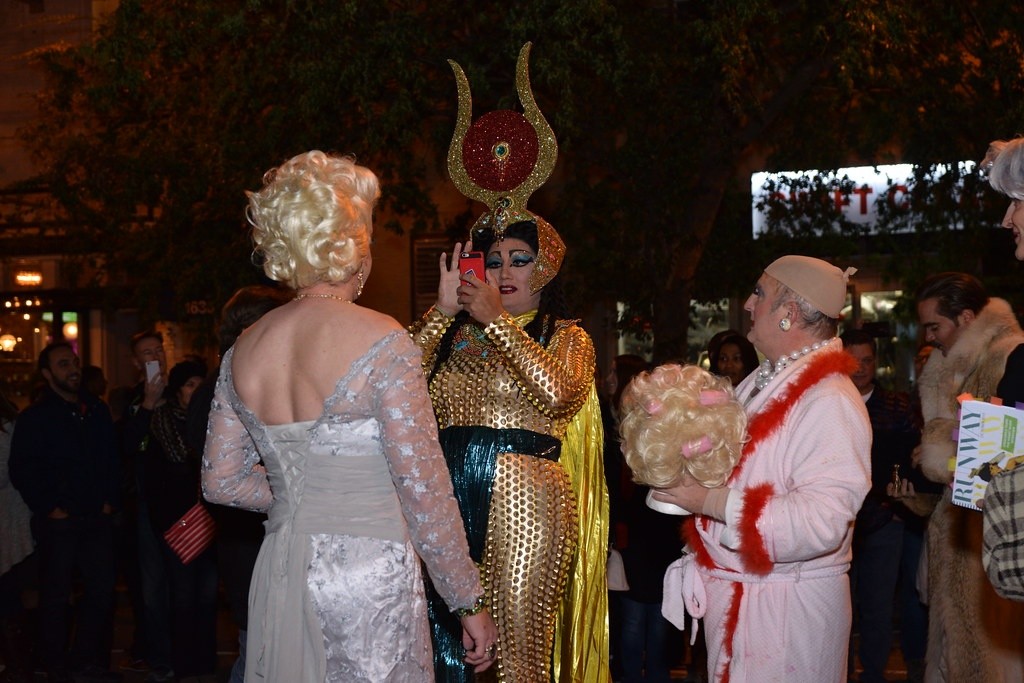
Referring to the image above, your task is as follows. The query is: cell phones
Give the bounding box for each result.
[144,360,160,385]
[457,250,485,297]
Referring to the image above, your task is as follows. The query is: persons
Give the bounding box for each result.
[201,150,500,683]
[841,330,930,683]
[596,330,760,683]
[408,209,610,683]
[886,137,1024,683]
[0,287,289,683]
[652,255,873,683]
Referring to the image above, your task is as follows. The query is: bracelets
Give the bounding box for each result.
[457,596,486,616]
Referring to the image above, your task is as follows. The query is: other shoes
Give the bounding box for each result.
[28,646,75,683]
[144,663,175,683]
[63,653,122,683]
[118,655,152,672]
[113,573,128,592]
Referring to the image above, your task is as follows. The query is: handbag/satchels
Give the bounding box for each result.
[162,502,220,567]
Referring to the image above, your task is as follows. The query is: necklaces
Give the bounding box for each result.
[753,337,836,389]
[295,294,352,303]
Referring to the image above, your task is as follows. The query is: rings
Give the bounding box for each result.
[486,641,497,659]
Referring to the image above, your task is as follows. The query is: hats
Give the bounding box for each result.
[184,354,209,378]
[169,362,202,390]
[764,255,859,319]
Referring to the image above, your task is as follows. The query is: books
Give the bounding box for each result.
[952,400,1024,512]
